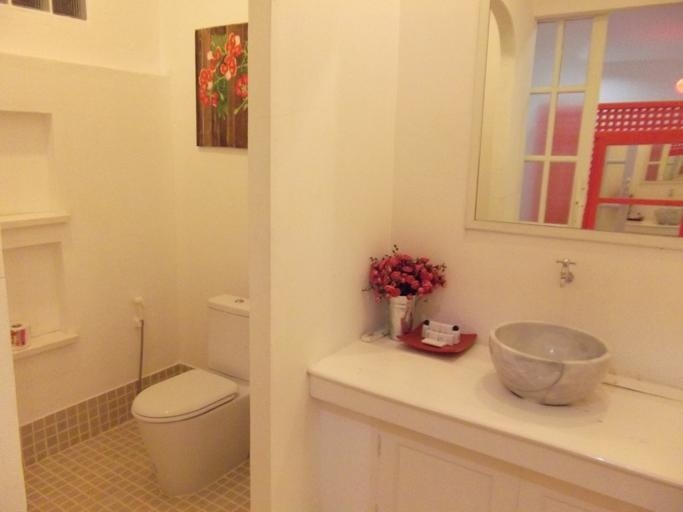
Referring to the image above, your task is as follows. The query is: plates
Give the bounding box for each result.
[397,321,478,356]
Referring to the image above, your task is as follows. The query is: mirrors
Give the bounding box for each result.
[462,0,682,257]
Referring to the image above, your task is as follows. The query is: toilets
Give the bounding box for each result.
[132,292,250,494]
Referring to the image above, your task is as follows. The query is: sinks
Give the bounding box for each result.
[489,319,613,405]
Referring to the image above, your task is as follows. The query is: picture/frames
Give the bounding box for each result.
[194,23,248,151]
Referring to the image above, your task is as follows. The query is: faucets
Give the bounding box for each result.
[557,258,578,288]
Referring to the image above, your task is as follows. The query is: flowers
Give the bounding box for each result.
[360,242,447,305]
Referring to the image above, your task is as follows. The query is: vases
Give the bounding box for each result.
[389,295,415,342]
